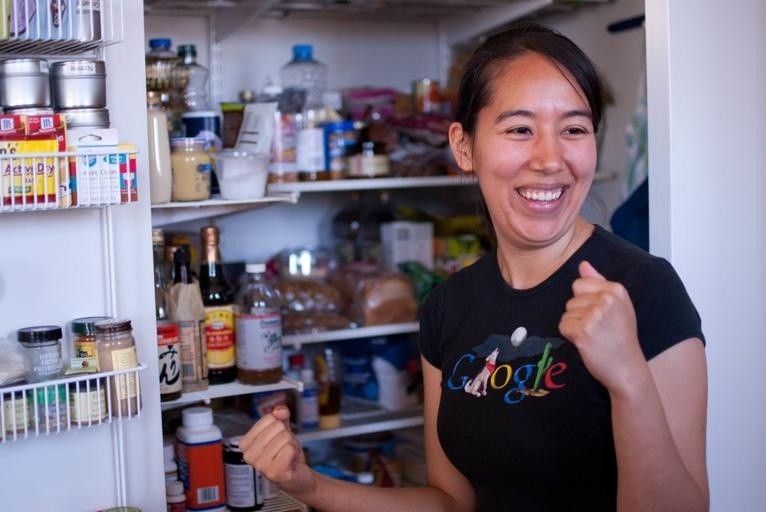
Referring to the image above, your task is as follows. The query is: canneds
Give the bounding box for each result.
[73,316,99,375]
[96,319,142,417]
[170,138,212,203]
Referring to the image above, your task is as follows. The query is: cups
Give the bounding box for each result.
[212,148,272,199]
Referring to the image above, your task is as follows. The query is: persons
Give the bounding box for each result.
[235,21,712,512]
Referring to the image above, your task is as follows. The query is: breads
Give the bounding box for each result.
[353,276,419,325]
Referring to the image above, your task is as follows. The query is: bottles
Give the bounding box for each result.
[237,261,284,386]
[152,222,184,404]
[218,43,391,179]
[284,343,345,431]
[144,36,220,202]
[197,227,238,384]
[168,237,210,393]
[162,407,226,510]
[0,313,141,431]
